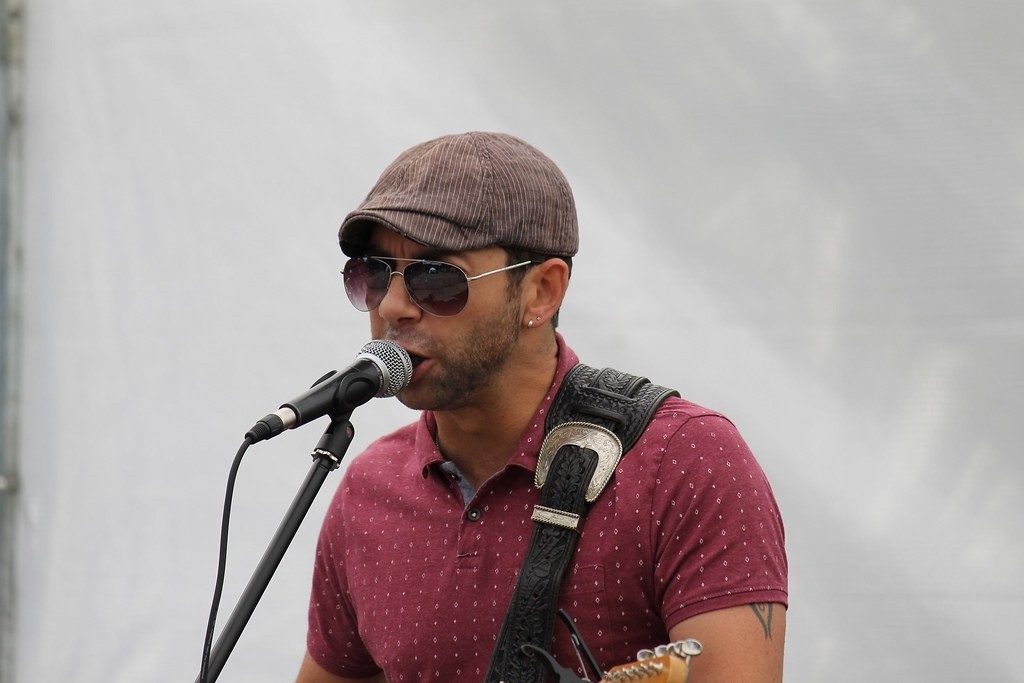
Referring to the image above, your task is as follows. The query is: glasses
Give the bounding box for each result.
[343,255,542,318]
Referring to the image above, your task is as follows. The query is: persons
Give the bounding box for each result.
[297,130,790,683]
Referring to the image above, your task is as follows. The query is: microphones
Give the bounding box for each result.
[245,340,413,444]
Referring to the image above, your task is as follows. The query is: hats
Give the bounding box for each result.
[337,130,579,257]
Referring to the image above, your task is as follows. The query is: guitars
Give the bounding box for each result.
[596,637,704,683]
